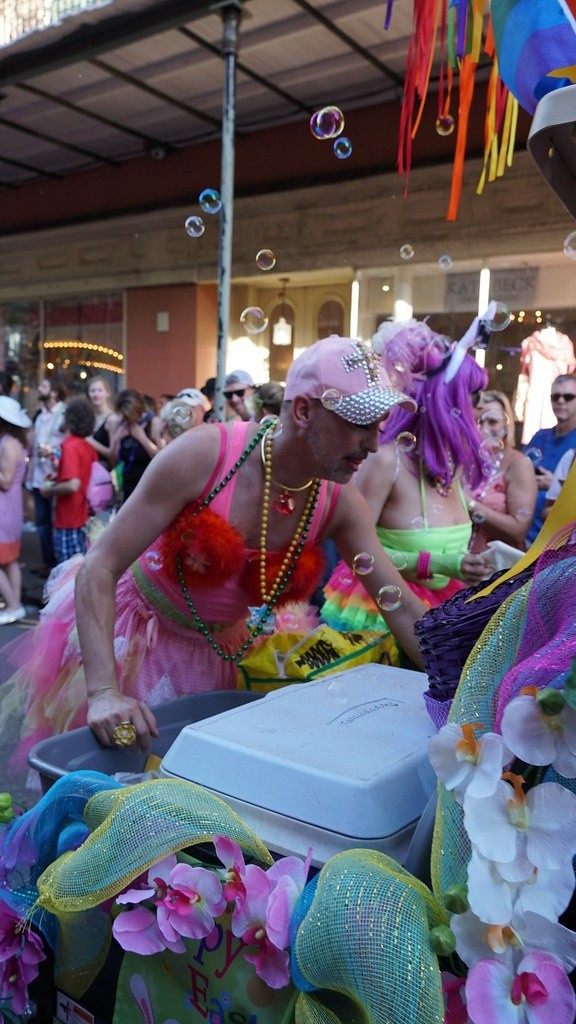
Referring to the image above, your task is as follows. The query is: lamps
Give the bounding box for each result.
[271,278,291,346]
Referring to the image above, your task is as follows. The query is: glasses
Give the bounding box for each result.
[550,393,576,402]
[224,386,252,400]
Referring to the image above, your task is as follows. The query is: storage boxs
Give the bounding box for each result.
[156,665,435,867]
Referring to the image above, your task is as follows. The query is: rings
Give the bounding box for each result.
[113,722,137,747]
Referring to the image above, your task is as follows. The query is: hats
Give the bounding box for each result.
[0,395,33,428]
[176,387,213,411]
[284,334,418,424]
[223,370,254,387]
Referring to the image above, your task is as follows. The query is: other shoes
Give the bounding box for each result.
[0,605,26,625]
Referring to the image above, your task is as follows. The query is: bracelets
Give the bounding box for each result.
[415,550,432,581]
[469,500,477,514]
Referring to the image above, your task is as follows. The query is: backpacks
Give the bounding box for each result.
[83,461,113,512]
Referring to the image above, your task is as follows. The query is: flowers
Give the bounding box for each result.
[0,682,576,1024]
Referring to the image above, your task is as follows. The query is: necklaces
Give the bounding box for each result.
[174,415,323,661]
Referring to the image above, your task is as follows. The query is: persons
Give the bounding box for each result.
[514,326,576,445]
[322,315,497,633]
[463,388,537,555]
[200,370,256,423]
[28,374,121,577]
[249,382,286,421]
[0,332,432,793]
[523,373,576,551]
[0,370,33,626]
[107,387,213,516]
[6,359,25,408]
